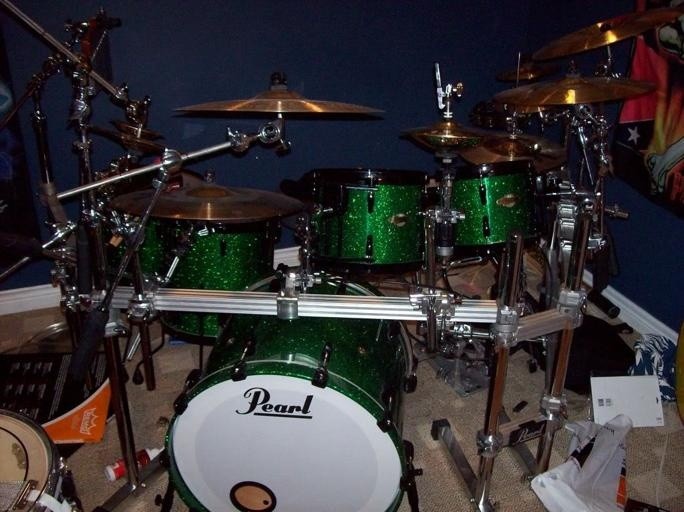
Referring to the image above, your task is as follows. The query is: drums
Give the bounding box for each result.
[431,159,540,260]
[139,218,274,337]
[166,271,409,512]
[306,169,426,270]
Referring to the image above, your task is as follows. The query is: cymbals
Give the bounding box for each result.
[530,8,681,61]
[398,122,487,145]
[108,182,304,220]
[505,64,568,84]
[460,131,566,169]
[496,80,654,105]
[175,91,386,115]
[89,119,169,154]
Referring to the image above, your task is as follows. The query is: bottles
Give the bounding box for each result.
[104,447,162,483]
